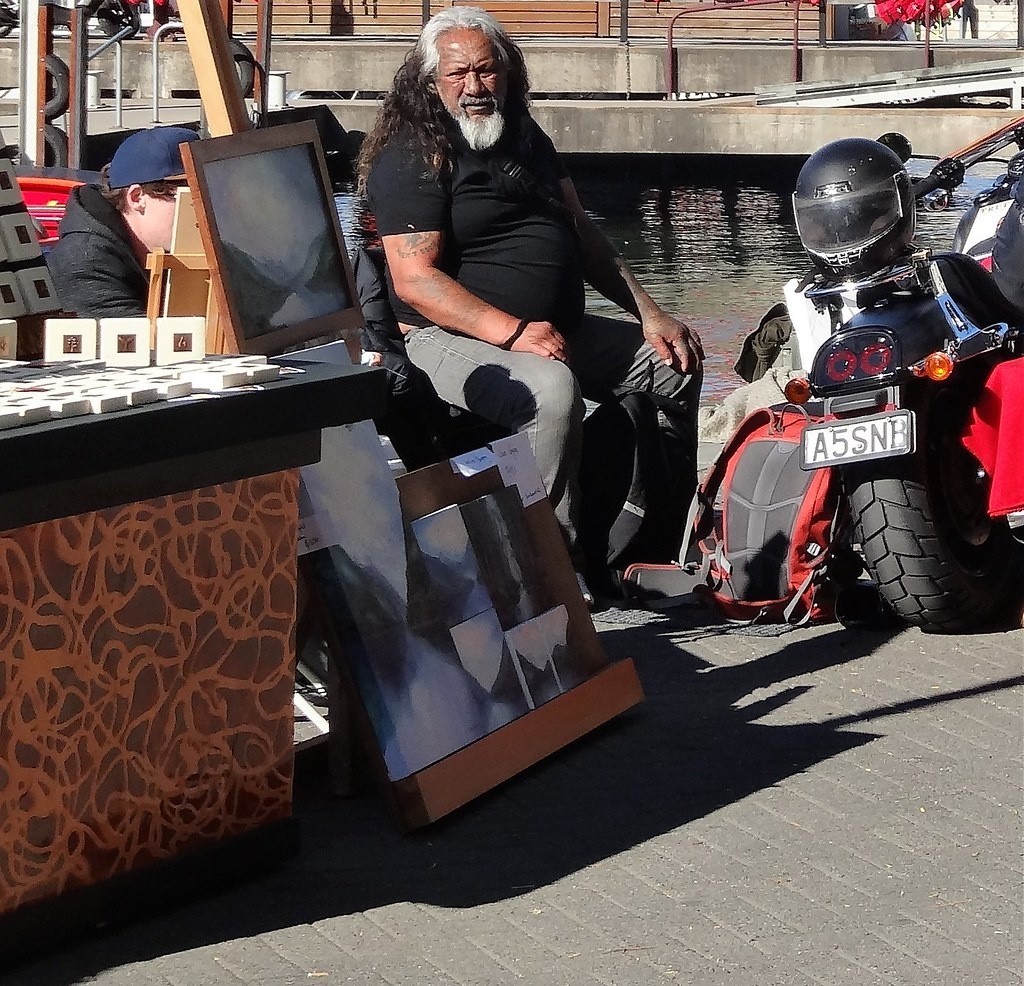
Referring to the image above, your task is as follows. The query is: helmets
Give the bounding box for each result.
[791,137,917,282]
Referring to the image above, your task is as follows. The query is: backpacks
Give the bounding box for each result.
[566,393,698,598]
[623,403,843,628]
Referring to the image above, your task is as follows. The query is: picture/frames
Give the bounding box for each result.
[178,119,367,358]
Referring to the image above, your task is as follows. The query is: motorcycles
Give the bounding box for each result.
[784,118,1023,634]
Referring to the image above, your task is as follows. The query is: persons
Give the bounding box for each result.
[46,128,200,318]
[358,6,706,605]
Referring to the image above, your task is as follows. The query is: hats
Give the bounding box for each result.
[108,126,201,189]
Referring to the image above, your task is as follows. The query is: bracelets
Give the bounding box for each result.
[501,317,530,351]
